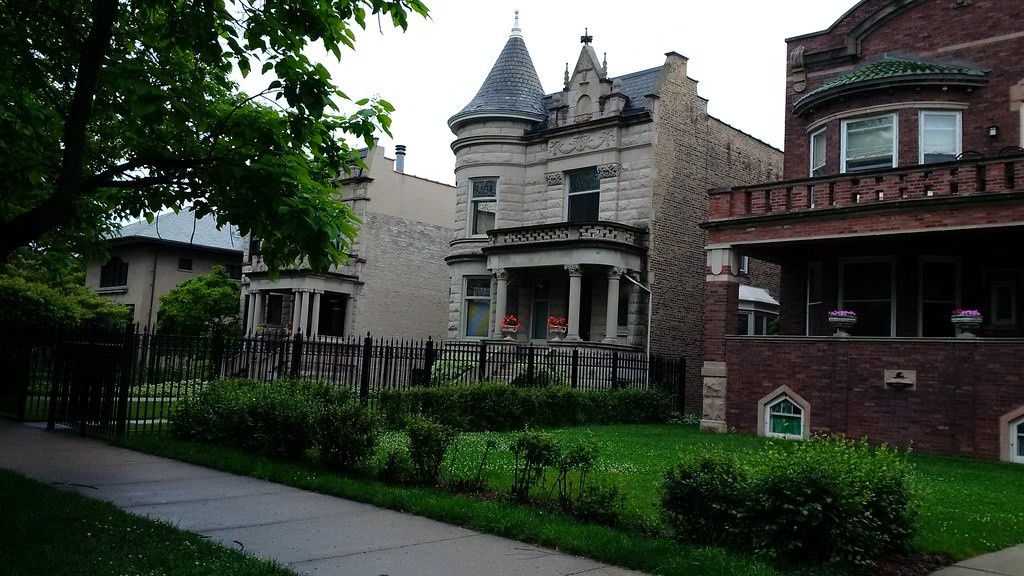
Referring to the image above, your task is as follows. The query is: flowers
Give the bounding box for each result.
[953,309,981,317]
[500,315,520,331]
[547,316,567,331]
[828,309,857,319]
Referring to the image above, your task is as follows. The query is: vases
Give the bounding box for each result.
[550,326,565,340]
[829,317,856,336]
[502,325,517,340]
[950,315,982,338]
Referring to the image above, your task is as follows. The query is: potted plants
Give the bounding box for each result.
[283,322,293,337]
[256,323,269,339]
